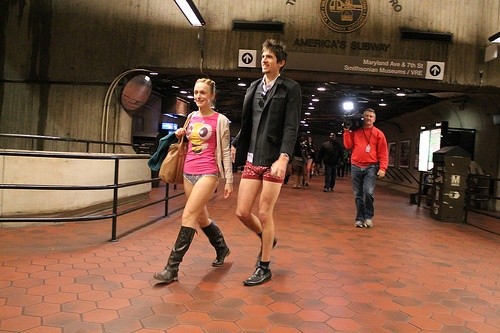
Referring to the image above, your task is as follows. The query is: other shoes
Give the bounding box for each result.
[306,182,308,187]
[331,188,334,191]
[292,184,300,188]
[302,181,304,185]
[324,188,328,191]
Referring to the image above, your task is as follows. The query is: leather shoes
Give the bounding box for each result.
[243,268,271,285]
[257,238,277,267]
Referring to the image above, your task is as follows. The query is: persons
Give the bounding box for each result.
[227,39,302,287]
[285,133,353,193]
[152,77,234,281]
[342,108,389,228]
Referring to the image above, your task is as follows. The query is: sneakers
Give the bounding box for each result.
[364,219,373,228]
[355,221,363,227]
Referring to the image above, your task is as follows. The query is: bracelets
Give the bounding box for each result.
[281,153,289,161]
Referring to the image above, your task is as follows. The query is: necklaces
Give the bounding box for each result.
[362,126,373,153]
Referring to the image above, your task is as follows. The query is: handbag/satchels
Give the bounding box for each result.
[158,116,193,184]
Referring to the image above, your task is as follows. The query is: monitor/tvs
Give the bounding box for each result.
[417,120,448,172]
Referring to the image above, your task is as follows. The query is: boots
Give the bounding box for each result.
[153,226,197,283]
[200,220,230,267]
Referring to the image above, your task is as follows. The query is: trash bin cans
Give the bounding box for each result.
[430,145,472,224]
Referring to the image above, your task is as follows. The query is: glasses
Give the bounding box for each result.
[259,91,268,109]
